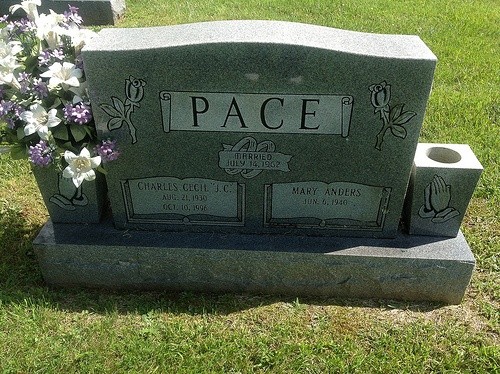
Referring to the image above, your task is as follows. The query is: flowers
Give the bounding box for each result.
[0,0,121,189]
